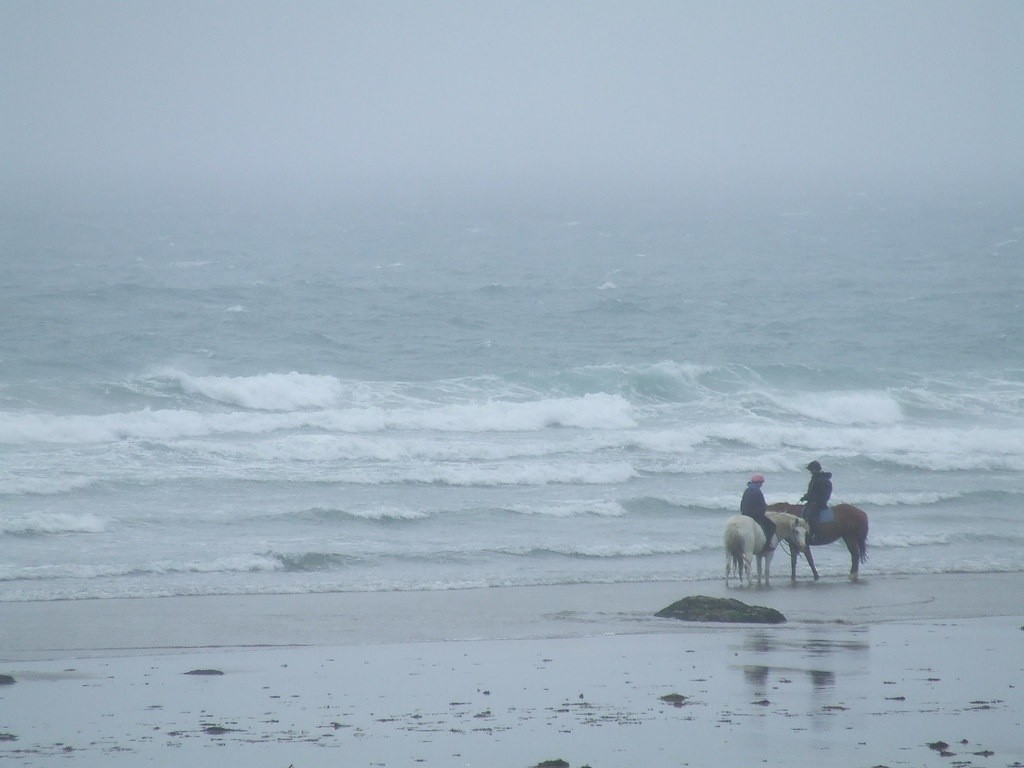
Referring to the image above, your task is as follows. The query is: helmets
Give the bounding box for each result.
[751,474,765,481]
[807,461,821,470]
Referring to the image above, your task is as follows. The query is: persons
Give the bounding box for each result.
[740,474,776,552]
[800,460,833,541]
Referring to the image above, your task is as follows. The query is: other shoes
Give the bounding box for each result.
[762,544,773,552]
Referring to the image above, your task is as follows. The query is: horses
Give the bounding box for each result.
[764,502,870,585]
[723,512,811,590]
